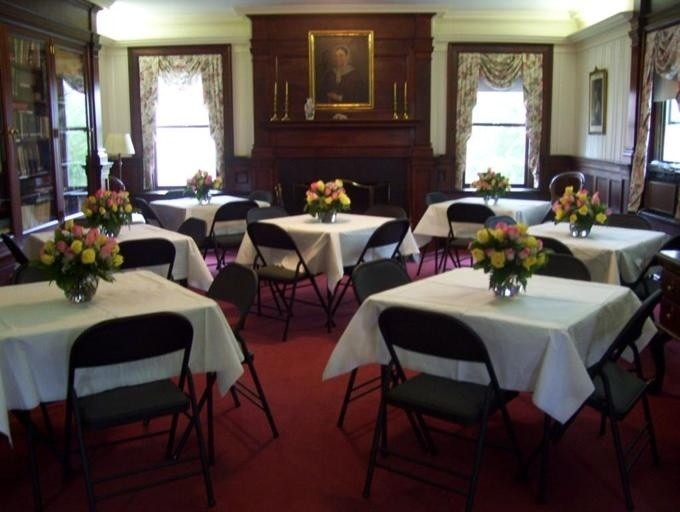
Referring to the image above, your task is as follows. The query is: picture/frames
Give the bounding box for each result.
[307,29,375,114]
[587,70,607,134]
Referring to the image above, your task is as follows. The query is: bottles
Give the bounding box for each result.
[305,98,315,120]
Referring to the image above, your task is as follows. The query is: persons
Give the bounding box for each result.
[323,47,362,103]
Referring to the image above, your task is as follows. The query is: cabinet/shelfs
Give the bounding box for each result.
[260,119,427,213]
[1,0,104,241]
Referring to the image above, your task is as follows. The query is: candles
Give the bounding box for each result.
[270,83,279,120]
[392,85,399,119]
[282,81,293,122]
[401,79,410,119]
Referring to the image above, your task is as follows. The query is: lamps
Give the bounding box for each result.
[104,132,135,180]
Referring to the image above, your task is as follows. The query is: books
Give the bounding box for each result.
[8,32,57,237]
